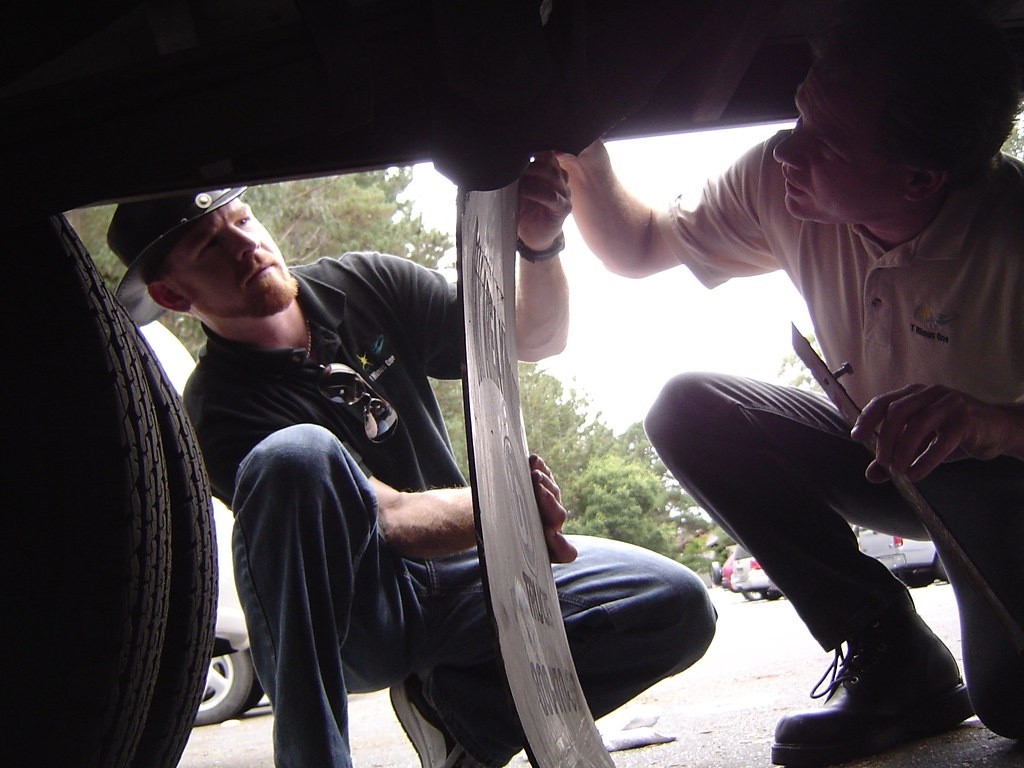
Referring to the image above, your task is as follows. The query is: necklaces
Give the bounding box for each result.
[302,330,312,358]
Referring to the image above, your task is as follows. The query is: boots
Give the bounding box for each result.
[772,585,975,765]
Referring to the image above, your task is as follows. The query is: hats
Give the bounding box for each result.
[106,186,249,326]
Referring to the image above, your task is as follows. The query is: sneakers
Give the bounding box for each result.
[389,674,481,768]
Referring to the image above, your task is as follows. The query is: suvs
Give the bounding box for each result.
[710,545,761,601]
[730,542,782,600]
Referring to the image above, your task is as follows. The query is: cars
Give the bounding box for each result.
[852,522,951,587]
[137,321,261,730]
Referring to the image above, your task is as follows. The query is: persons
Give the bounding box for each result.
[107,153,719,768]
[558,1,1024,768]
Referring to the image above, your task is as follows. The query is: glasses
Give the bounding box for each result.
[316,361,398,443]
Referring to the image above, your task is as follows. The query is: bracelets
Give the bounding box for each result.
[516,230,566,264]
